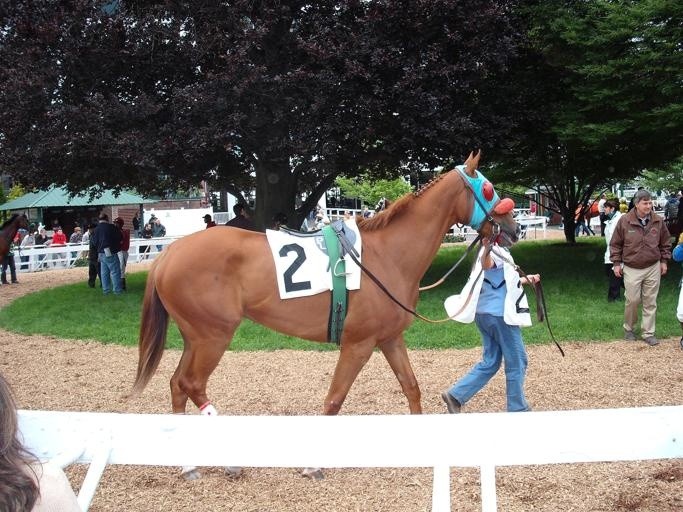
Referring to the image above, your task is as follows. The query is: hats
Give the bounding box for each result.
[113,218,124,224]
[202,214,210,218]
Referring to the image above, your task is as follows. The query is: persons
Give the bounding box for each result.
[0,373,44,511]
[561,188,683,348]
[441,236,541,412]
[269,212,298,233]
[0,204,254,296]
[530,200,537,226]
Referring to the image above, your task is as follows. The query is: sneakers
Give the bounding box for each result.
[442,393,460,414]
[625,331,636,341]
[645,336,659,345]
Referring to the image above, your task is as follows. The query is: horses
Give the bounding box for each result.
[375,197,392,212]
[562,199,599,236]
[0,212,35,289]
[120,149,521,481]
